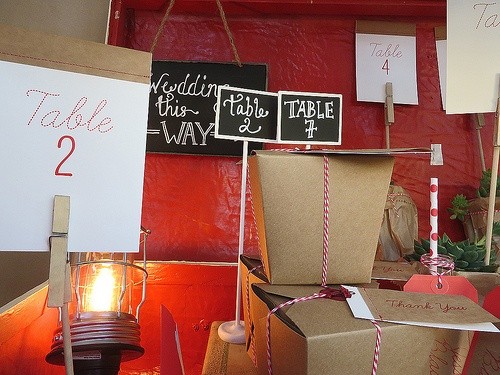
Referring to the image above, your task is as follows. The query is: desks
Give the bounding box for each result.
[200,319,251,374]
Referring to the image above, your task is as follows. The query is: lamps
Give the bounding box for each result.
[45,226,151,365]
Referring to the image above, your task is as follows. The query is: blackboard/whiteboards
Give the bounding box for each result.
[216,85,279,143]
[277,92,343,145]
[145,59,269,157]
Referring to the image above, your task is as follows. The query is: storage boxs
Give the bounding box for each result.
[247,149,395,285]
[249,283,475,375]
[240,254,380,368]
